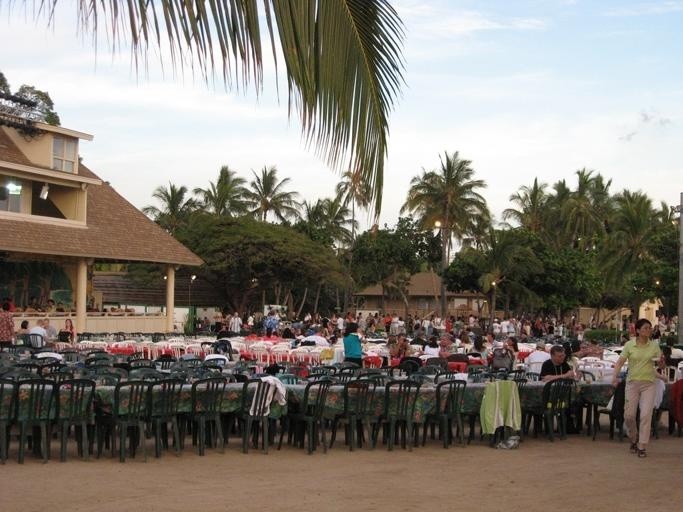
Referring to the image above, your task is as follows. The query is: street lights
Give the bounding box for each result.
[188,275,198,313]
[489,280,497,316]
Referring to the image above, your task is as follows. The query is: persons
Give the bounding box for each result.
[193,295,683,422]
[0,296,132,358]
[610,318,667,458]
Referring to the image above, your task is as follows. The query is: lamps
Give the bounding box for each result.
[39,182,49,200]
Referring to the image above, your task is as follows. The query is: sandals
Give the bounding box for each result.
[638,448,649,457]
[630,439,639,453]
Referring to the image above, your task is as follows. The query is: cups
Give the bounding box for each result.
[652,357,659,369]
[585,377,591,384]
